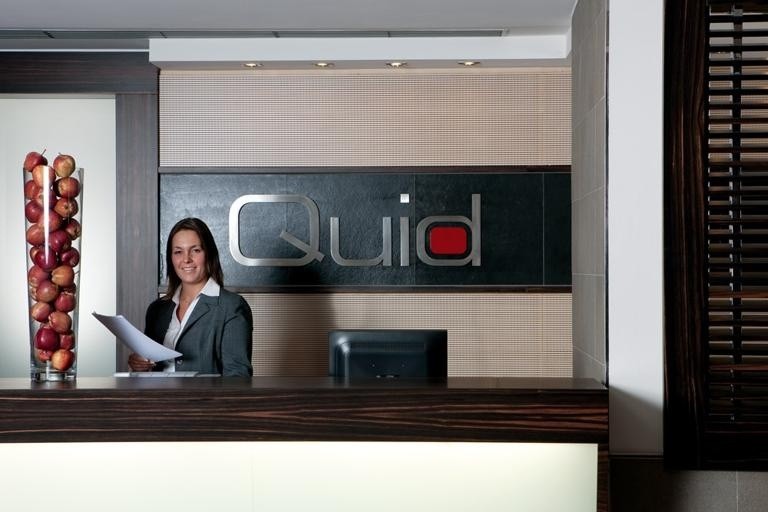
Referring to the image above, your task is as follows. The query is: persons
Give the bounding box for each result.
[126,216,255,376]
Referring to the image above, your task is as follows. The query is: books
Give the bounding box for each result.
[91,311,183,364]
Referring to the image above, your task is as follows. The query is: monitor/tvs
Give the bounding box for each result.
[326,329,448,379]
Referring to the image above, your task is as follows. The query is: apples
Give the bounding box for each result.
[23,149,82,372]
